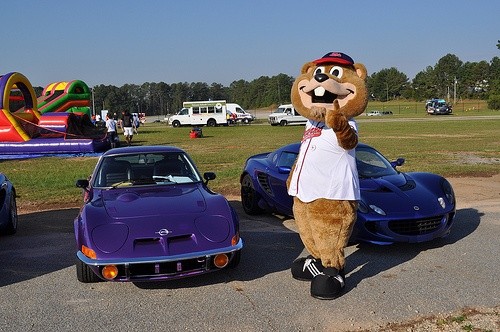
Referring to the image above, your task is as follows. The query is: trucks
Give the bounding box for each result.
[168,99,228,127]
[227,103,254,124]
[268,105,309,126]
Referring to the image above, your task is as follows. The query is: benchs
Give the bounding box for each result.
[129,162,155,180]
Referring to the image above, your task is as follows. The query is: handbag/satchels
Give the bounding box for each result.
[114,137,119,142]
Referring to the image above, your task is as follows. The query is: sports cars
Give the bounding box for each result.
[73,146,243,283]
[240,142,456,251]
[0,174,19,245]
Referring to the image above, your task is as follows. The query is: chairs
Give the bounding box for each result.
[98,160,130,187]
[153,157,188,182]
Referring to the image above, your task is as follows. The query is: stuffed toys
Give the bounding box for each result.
[286,52,369,300]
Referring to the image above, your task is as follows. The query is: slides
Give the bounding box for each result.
[14,80,91,113]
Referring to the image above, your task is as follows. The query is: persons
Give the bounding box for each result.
[96,112,100,121]
[106,114,118,143]
[120,109,137,146]
[227,113,237,123]
[189,126,202,138]
[91,114,95,120]
[134,115,138,129]
[113,112,118,128]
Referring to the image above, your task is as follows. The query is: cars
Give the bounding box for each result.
[367,110,380,117]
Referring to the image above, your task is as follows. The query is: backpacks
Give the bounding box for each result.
[123,116,130,126]
[135,120,140,128]
